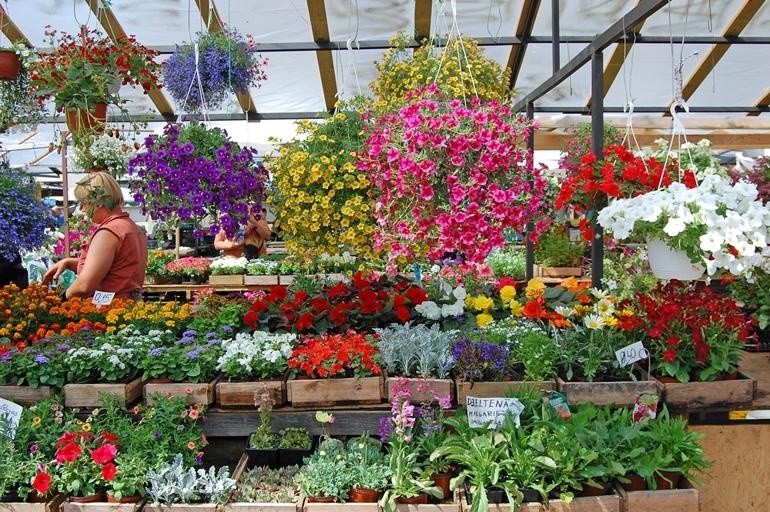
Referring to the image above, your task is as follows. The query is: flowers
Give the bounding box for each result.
[292,330,375,376]
[2,337,69,384]
[0,24,769,323]
[456,320,549,380]
[0,401,617,509]
[531,278,628,368]
[617,277,760,361]
[66,326,143,378]
[376,321,454,380]
[148,329,215,380]
[214,327,295,380]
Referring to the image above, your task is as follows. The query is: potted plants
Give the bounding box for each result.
[618,403,707,512]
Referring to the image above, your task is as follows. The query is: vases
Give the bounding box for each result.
[60,379,143,410]
[555,368,655,404]
[643,369,758,402]
[283,372,384,404]
[144,382,215,408]
[455,374,555,409]
[217,382,280,407]
[385,374,455,408]
[2,385,53,401]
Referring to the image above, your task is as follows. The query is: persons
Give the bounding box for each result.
[42,171,148,305]
[241,208,270,261]
[214,207,267,261]
